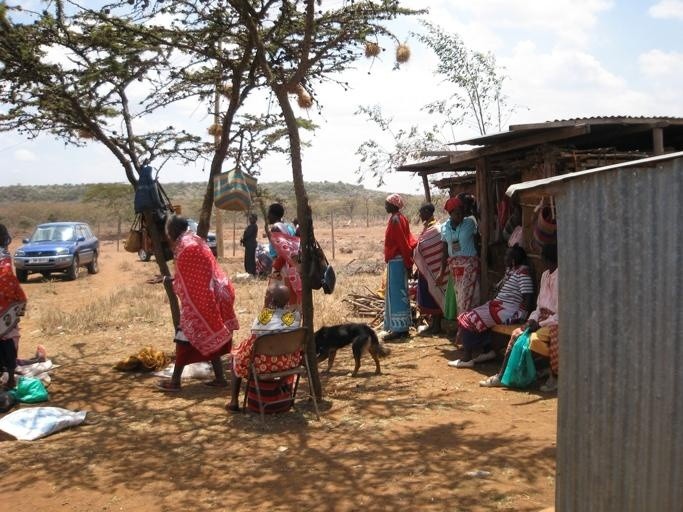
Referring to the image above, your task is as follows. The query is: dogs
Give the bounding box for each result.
[314,322,390,377]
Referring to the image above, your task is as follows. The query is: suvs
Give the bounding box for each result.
[13,221,100,281]
[137,217,216,261]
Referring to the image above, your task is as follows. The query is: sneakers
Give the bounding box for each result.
[448,350,502,387]
[541,376,558,392]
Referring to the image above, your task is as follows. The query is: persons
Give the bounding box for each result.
[144,214,239,392]
[291,216,301,237]
[0,224,27,391]
[381,192,560,393]
[223,285,301,414]
[267,203,303,320]
[268,218,295,260]
[239,213,259,277]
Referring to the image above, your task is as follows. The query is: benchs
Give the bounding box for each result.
[489,323,520,335]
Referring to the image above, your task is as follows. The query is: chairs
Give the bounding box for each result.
[242,326,321,431]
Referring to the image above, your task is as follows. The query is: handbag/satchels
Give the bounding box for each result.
[321,265,336,295]
[124,164,182,261]
[213,166,257,210]
[306,247,328,290]
[248,378,292,414]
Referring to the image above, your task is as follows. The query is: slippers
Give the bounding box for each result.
[225,403,239,413]
[203,379,230,390]
[383,332,409,340]
[417,327,444,337]
[154,380,181,391]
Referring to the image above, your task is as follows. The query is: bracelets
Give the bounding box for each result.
[162,275,167,285]
